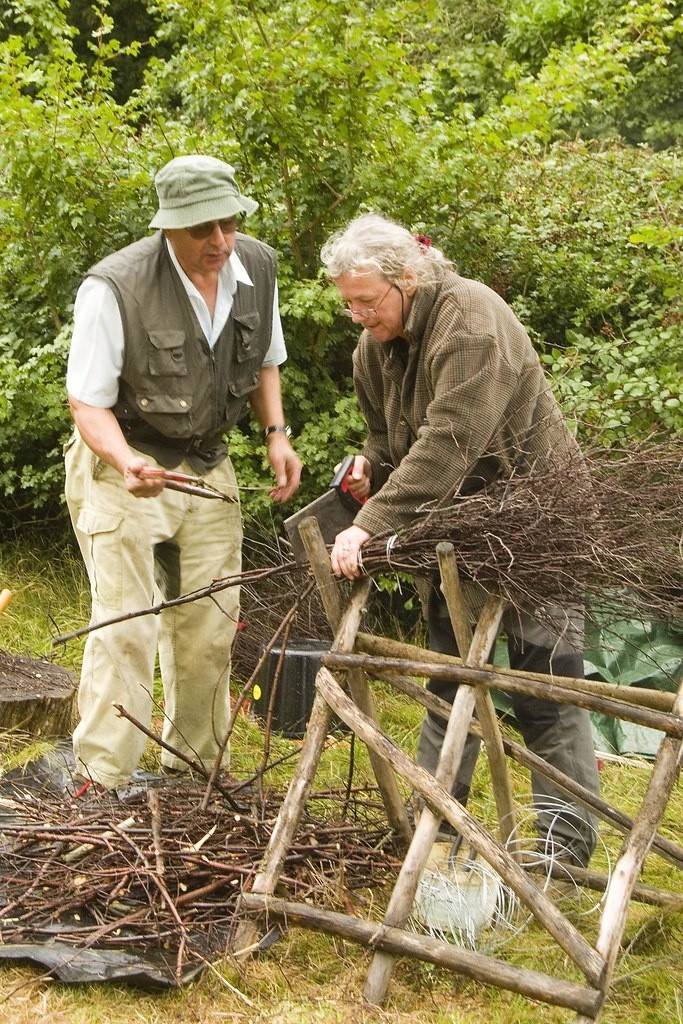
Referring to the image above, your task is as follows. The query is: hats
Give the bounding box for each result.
[147,154,259,229]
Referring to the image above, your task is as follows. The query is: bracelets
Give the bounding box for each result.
[258,424,293,445]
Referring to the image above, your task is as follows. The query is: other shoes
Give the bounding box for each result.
[89,780,146,804]
[159,764,255,796]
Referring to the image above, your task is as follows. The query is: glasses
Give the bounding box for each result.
[341,284,393,319]
[185,216,237,240]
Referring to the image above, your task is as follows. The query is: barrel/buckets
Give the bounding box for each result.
[411,841,500,933]
[254,634,356,739]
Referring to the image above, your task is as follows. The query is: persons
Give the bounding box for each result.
[317,215,602,908]
[69,154,303,800]
